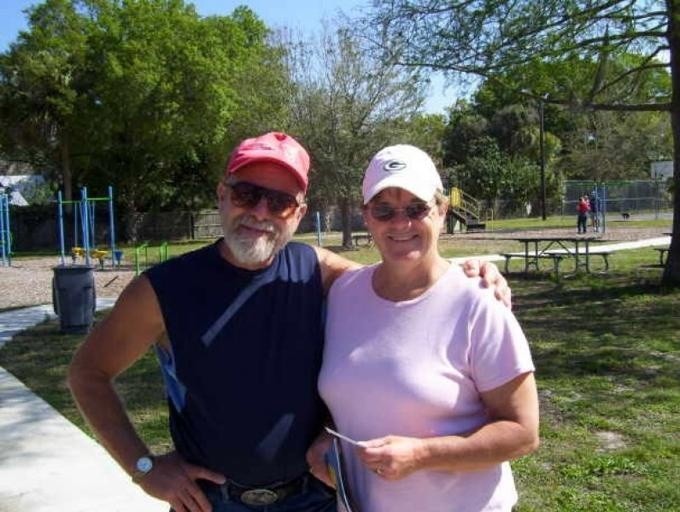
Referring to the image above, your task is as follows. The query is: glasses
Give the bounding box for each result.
[370,201,438,222]
[225,181,307,219]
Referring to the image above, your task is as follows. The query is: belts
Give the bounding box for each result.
[205,472,319,507]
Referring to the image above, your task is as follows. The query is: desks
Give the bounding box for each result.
[661,232,673,248]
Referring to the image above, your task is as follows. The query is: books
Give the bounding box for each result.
[324,435,353,512]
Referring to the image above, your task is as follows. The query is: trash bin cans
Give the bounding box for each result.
[53,265,97,333]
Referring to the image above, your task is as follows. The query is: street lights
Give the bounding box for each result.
[520,87,548,223]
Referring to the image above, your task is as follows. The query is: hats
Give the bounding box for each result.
[363,144,444,206]
[225,131,310,194]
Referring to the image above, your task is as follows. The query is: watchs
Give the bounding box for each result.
[130,450,159,485]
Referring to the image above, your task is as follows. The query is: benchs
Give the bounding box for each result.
[352,235,372,246]
[654,248,673,266]
[495,234,614,282]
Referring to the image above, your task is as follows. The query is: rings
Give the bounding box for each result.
[308,464,315,475]
[374,461,385,475]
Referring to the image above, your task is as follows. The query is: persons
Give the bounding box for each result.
[65,129,513,512]
[589,191,603,233]
[576,195,592,234]
[304,142,541,511]
